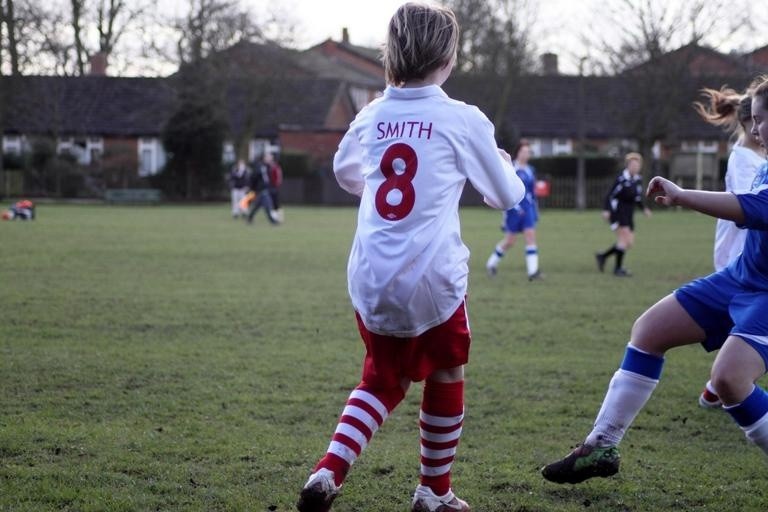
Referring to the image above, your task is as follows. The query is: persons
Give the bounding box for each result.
[2,201,37,223]
[483,137,548,283]
[223,149,284,224]
[291,0,526,512]
[593,149,653,277]
[692,78,768,408]
[537,76,767,485]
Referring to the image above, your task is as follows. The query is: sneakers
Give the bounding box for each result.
[297,468,342,512]
[528,268,546,283]
[614,267,634,277]
[409,483,471,512]
[593,250,606,274]
[485,265,498,277]
[539,443,621,485]
[697,379,723,408]
